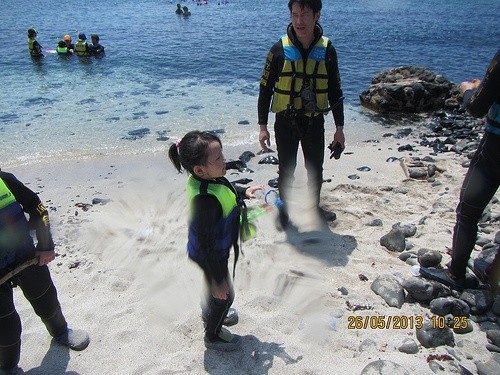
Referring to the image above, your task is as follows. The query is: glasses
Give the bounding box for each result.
[302,89,320,112]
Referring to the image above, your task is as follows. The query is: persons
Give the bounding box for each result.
[27,28,44,57]
[419,50,500,290]
[257,0,345,231]
[56,33,105,54]
[175,4,191,16]
[168,130,263,351]
[0,169,89,375]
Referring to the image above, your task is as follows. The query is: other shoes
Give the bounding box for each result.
[55,328,89,350]
[419,266,463,291]
[474,258,493,282]
[317,205,336,221]
[275,211,288,231]
[223,308,238,324]
[204,332,242,351]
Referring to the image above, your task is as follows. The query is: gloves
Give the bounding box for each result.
[328,141,345,160]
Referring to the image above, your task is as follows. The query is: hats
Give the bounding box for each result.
[79,33,87,40]
[64,35,70,41]
